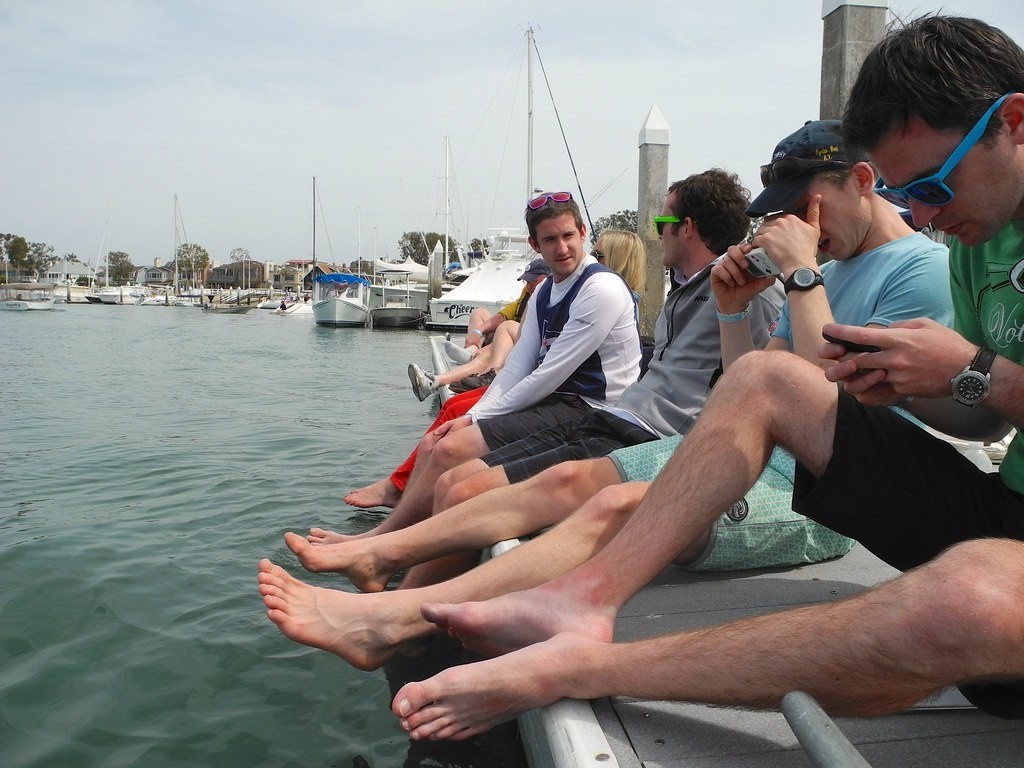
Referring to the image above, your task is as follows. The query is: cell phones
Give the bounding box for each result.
[743,246,781,278]
[821,332,882,353]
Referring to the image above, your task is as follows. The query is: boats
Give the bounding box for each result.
[312,273,369,327]
[65,298,93,304]
[257,298,287,310]
[370,272,424,329]
[0,283,58,310]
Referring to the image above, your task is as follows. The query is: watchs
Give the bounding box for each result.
[784,268,825,295]
[949,347,997,408]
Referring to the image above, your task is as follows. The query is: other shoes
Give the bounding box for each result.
[449,368,497,394]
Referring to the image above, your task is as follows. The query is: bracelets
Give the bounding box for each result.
[473,329,483,337]
[716,302,750,322]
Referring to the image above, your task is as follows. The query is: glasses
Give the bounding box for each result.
[761,156,853,188]
[590,251,605,260]
[527,192,574,211]
[873,89,1019,210]
[653,216,700,236]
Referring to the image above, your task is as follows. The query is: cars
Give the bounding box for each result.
[76,280,87,286]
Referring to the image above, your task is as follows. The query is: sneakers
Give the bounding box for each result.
[408,363,435,402]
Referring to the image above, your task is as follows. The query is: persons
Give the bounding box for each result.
[306,192,642,546]
[392,17,1024,739]
[344,228,646,507]
[408,259,552,402]
[259,121,955,670]
[397,168,785,659]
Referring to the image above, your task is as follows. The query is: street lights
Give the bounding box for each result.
[356,204,361,277]
[373,226,378,284]
[133,271,137,289]
[303,255,306,291]
[242,249,250,290]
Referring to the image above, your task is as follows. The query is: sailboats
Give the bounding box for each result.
[84,192,205,308]
[271,23,598,332]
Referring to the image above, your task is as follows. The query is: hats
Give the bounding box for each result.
[745,119,871,218]
[517,258,552,282]
